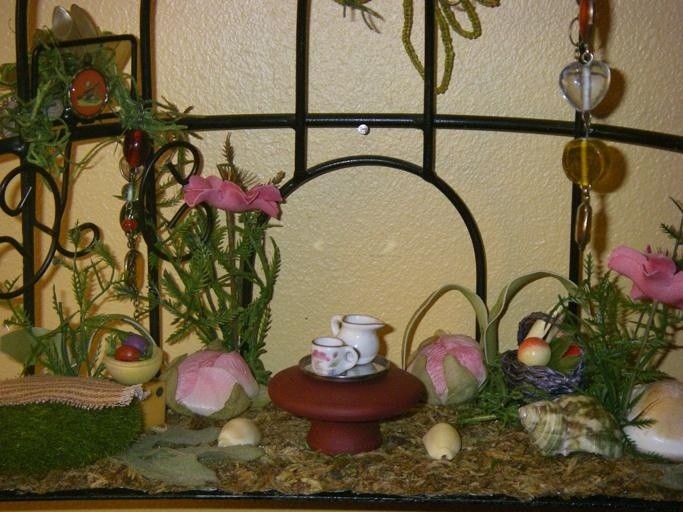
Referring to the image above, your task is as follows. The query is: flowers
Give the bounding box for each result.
[177,170,285,350]
[602,238,683,430]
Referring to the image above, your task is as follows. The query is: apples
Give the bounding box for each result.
[518,337,552,366]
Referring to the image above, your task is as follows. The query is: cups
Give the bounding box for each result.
[311,337,357,377]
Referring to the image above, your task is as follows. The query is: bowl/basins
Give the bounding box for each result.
[104,345,164,385]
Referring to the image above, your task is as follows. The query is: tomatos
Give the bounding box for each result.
[116,346,145,362]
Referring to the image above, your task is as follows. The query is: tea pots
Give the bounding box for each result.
[331,313,387,366]
